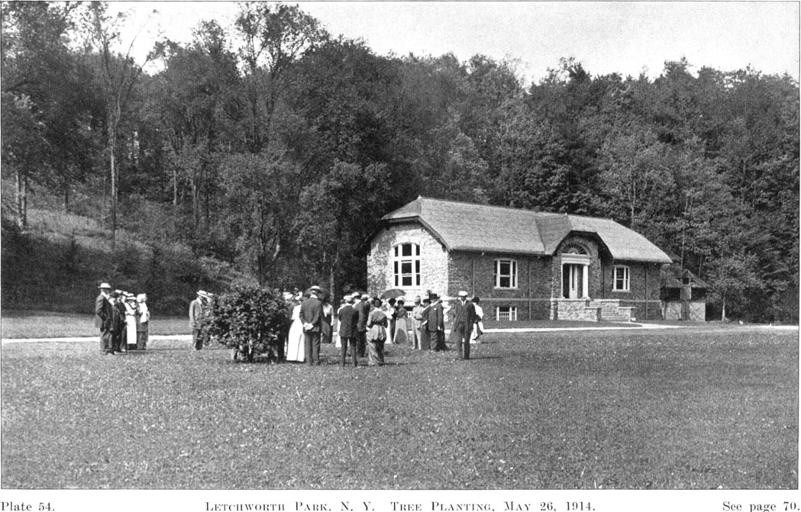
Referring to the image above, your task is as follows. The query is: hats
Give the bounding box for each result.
[98,282,148,302]
[471,296,480,302]
[459,290,469,298]
[196,289,216,301]
[414,293,439,304]
[343,291,405,308]
[295,286,323,301]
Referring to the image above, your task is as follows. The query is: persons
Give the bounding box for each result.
[189,289,218,350]
[94,283,150,356]
[273,285,484,367]
